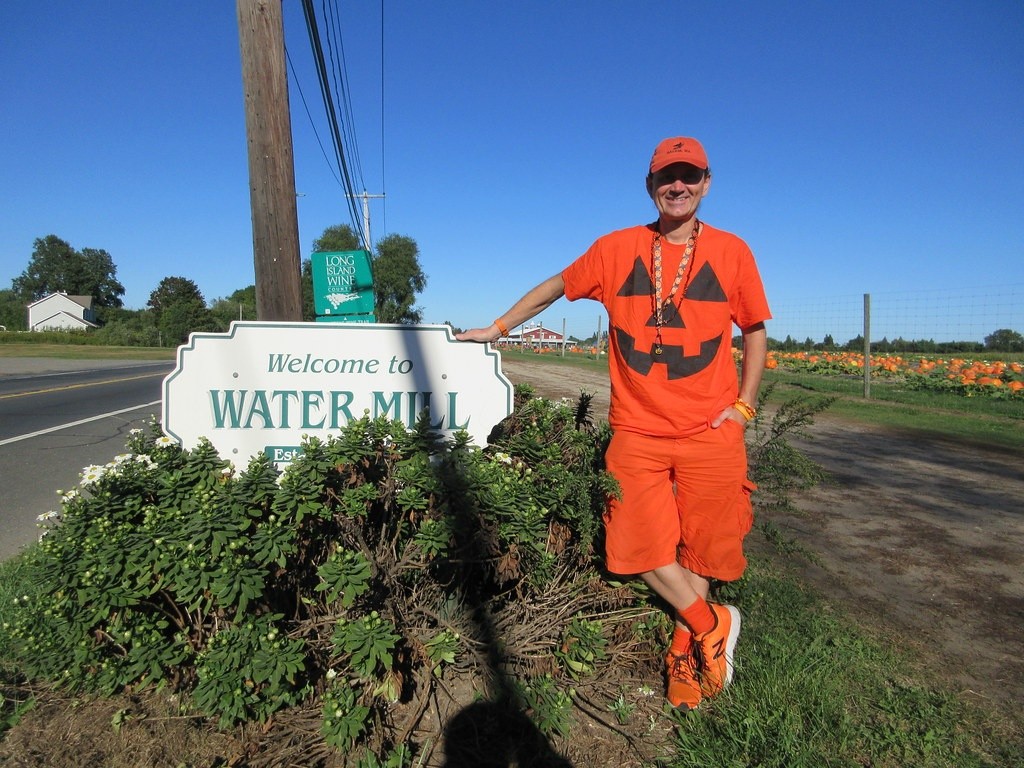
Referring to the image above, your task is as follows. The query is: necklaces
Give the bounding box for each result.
[649,216,700,354]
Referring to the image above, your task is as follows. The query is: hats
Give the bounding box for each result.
[650,137,708,174]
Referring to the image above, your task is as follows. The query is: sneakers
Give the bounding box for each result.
[697,601,742,698]
[665,646,702,713]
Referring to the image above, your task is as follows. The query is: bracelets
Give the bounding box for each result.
[735,398,756,416]
[734,403,751,422]
[494,318,509,338]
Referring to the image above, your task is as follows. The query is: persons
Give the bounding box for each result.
[455,134,772,714]
